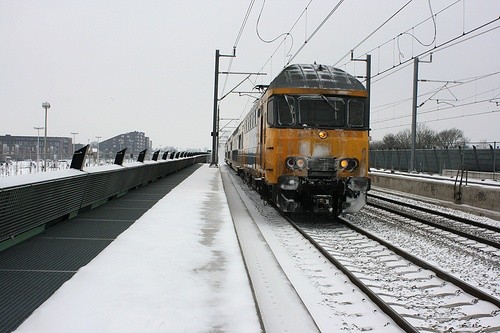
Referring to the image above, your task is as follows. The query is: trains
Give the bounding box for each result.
[223,61,371,216]
